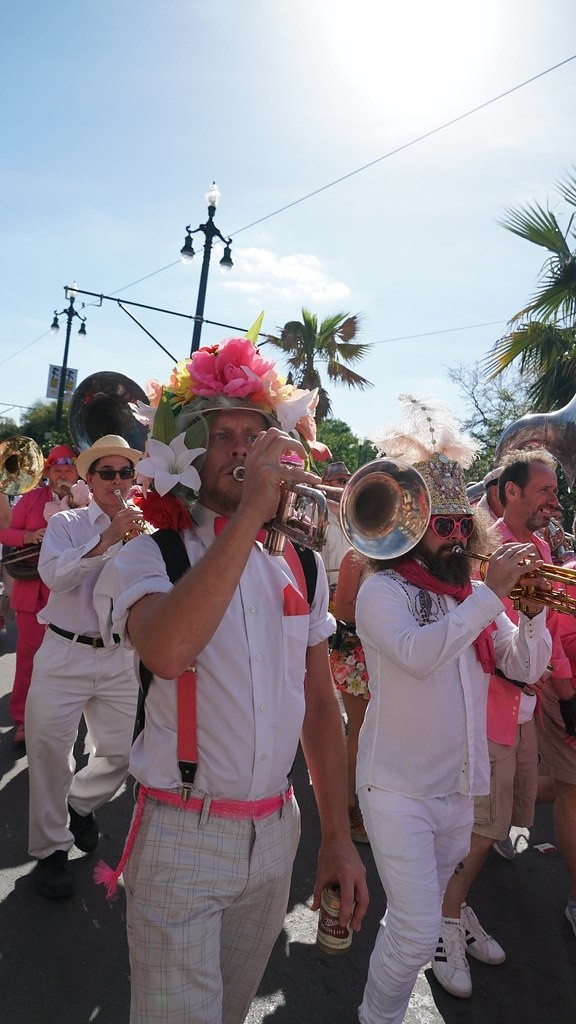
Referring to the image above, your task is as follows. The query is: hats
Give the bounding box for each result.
[43,445,78,478]
[483,465,505,489]
[179,398,281,430]
[76,435,142,480]
[321,462,352,481]
[374,393,481,515]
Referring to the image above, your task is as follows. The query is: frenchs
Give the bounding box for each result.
[0,371,150,579]
[495,393,576,567]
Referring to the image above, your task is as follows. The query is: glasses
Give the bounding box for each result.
[428,516,476,539]
[91,467,135,480]
[330,478,347,484]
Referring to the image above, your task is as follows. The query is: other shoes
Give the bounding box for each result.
[15,724,25,741]
[67,803,99,851]
[347,804,369,842]
[494,836,514,860]
[36,850,75,898]
[564,903,576,932]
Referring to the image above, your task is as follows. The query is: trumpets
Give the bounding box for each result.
[452,544,576,619]
[113,488,150,544]
[0,434,45,497]
[233,457,433,560]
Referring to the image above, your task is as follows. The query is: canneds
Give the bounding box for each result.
[521,664,554,696]
[317,883,352,956]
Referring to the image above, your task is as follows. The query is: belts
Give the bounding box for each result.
[47,623,120,648]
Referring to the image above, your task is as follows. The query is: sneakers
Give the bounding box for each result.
[431,917,472,997]
[459,901,506,963]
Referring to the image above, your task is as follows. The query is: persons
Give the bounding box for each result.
[0,310,371,1024]
[303,390,576,1024]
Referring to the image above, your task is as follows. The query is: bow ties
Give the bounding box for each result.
[214,516,267,544]
